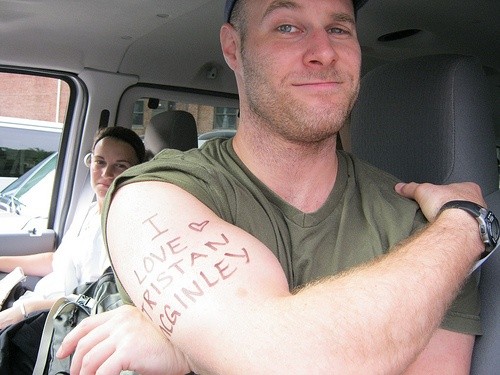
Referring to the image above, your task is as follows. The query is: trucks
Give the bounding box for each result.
[0,129,236,232]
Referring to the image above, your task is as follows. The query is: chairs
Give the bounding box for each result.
[144,56,500,375]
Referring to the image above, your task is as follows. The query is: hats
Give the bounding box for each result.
[222,0,368,27]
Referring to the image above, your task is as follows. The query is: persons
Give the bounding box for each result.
[0,126,145,331]
[54,0,500,374]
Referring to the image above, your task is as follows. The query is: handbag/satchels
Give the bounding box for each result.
[32,266,125,375]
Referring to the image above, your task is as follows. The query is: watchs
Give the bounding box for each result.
[435,200,500,260]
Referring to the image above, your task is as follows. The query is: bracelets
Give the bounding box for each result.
[20,299,29,319]
